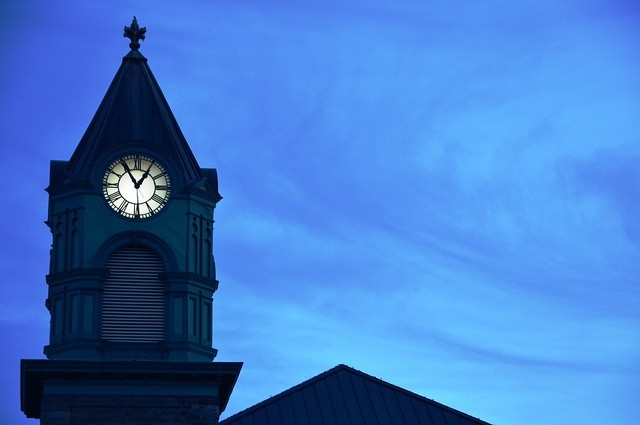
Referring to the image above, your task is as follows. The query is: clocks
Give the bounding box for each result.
[101,150,173,223]
